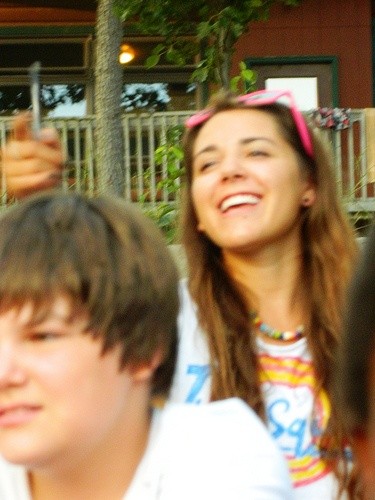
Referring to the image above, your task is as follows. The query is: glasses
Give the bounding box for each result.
[182,88,316,158]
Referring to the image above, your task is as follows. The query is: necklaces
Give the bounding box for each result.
[243,307,308,341]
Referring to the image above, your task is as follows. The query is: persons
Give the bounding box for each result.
[0,190,295,500]
[3,88,375,500]
